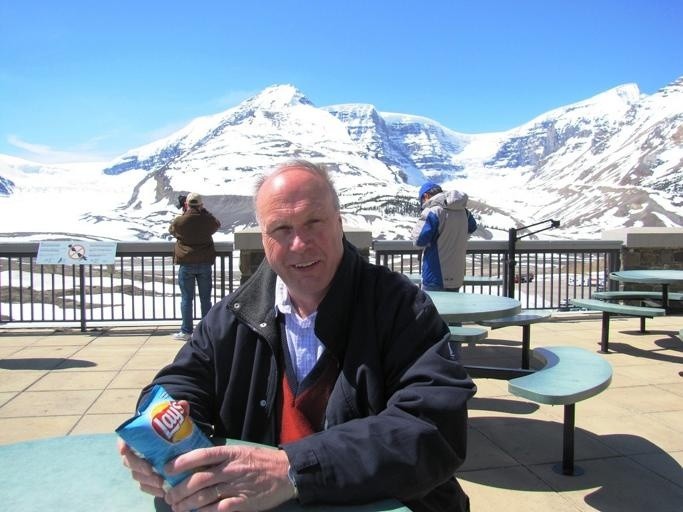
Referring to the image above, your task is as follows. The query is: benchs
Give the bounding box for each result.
[567,290,682,353]
[438,309,611,478]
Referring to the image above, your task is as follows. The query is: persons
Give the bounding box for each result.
[116,160,477,512]
[169,192,221,341]
[412,182,477,361]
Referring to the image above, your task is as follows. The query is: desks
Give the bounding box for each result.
[420,284,520,384]
[1,431,412,512]
[608,269,683,318]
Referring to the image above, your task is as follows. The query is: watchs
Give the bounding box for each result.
[288,463,299,499]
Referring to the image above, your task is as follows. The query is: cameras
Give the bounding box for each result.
[175,194,186,209]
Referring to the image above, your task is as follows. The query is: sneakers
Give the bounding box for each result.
[171,331,192,341]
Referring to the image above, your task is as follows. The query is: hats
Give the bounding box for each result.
[186,191,203,208]
[418,182,441,212]
